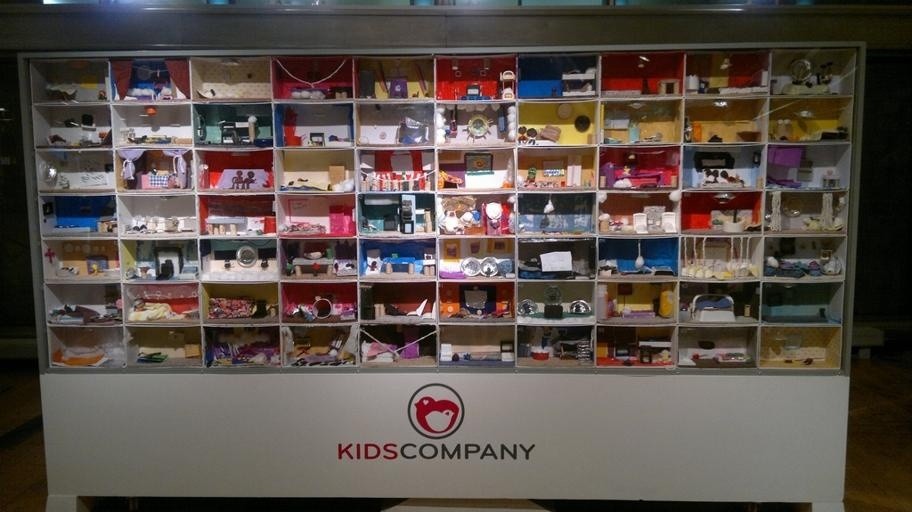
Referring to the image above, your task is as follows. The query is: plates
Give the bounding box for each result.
[481,259,500,275]
[557,103,572,117]
[461,257,478,275]
[236,246,256,265]
[468,115,489,138]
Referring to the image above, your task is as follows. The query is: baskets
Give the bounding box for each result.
[687,293,735,323]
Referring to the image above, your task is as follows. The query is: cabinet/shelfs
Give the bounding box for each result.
[16,42,866,512]
